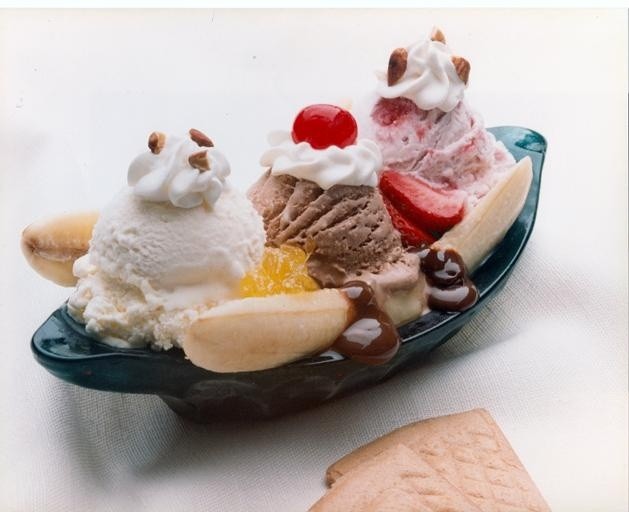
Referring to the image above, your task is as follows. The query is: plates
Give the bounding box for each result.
[24,122,547,430]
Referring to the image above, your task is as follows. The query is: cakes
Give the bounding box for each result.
[16,26,536,375]
